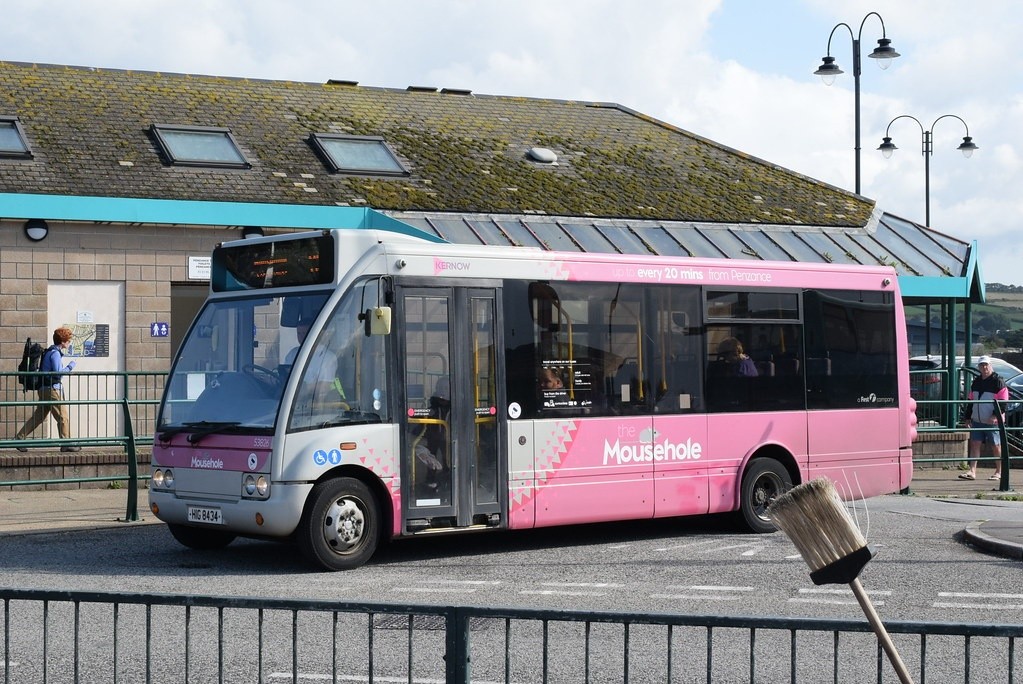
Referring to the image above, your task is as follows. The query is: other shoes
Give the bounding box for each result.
[412,482,439,495]
[12,437,28,451]
[60,446,81,452]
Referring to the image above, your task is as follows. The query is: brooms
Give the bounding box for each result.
[760,473,913,683]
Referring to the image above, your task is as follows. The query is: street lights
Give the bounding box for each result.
[877,114,980,229]
[812,12,901,196]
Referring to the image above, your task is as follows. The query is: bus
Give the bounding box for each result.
[145,228,920,571]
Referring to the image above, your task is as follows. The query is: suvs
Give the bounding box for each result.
[908,354,1023,428]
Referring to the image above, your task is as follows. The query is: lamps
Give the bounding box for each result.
[242,226,264,239]
[24,220,49,242]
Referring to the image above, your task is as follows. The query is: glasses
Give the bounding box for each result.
[979,362,989,366]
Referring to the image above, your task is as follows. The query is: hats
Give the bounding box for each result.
[978,355,991,363]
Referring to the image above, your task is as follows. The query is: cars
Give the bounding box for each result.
[998,373,1023,440]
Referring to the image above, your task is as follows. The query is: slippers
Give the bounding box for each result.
[958,472,976,480]
[988,473,1000,480]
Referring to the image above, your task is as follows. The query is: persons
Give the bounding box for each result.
[12,327,81,452]
[718,337,759,378]
[539,365,570,406]
[420,374,450,485]
[283,317,339,405]
[958,355,1009,481]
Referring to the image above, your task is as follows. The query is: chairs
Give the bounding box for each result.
[614,332,884,399]
[328,340,345,395]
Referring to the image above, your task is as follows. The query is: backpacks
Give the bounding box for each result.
[18,337,62,392]
